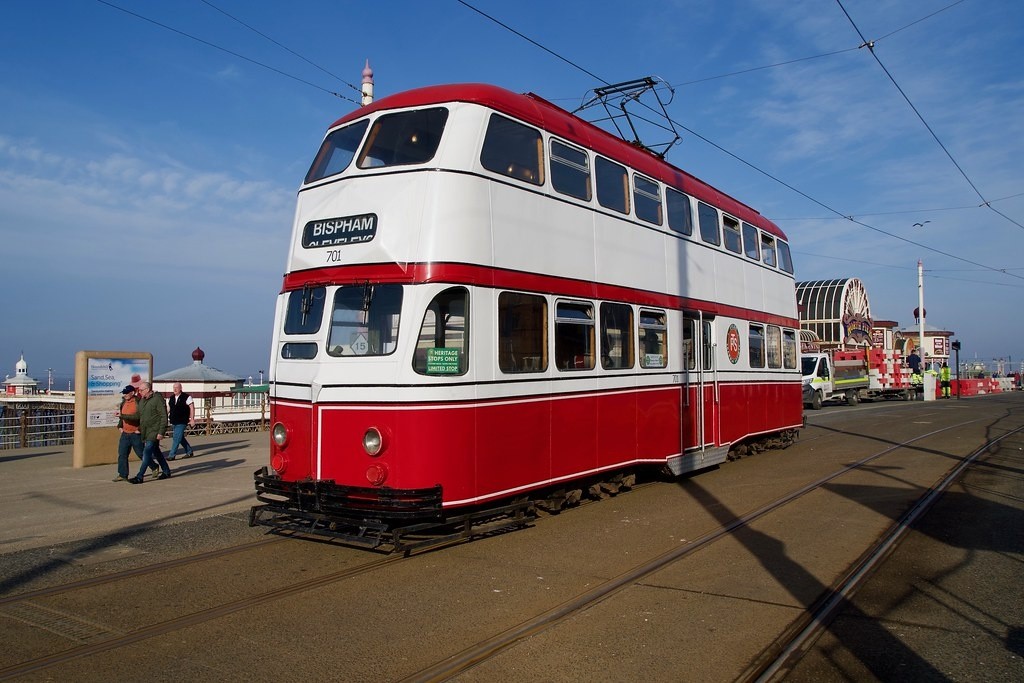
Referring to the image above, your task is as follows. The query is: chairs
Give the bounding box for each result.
[507,165,784,268]
[415,345,666,376]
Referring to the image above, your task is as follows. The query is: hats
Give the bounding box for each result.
[119,385,135,394]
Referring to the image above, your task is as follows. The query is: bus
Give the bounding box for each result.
[246,81,807,558]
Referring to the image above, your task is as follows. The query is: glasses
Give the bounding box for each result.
[139,388,147,391]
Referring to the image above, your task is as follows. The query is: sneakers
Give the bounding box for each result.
[152,465,158,478]
[113,476,128,481]
[158,474,170,480]
[128,476,143,483]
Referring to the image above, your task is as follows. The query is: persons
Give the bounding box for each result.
[938,361,952,400]
[110,385,160,482]
[113,382,169,485]
[905,349,923,386]
[992,370,1001,379]
[1014,369,1020,386]
[978,370,985,378]
[1019,370,1024,391]
[164,382,196,461]
[1007,370,1014,378]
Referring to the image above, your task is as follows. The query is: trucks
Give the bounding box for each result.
[801,352,870,409]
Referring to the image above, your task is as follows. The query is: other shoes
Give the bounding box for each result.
[182,453,194,459]
[166,457,175,460]
